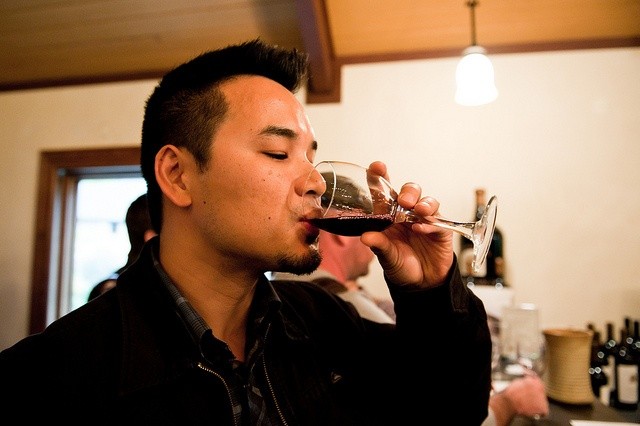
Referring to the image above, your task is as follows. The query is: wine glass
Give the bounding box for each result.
[299,161,499,269]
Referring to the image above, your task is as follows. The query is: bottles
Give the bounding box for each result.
[630,320,636,357]
[581,324,603,398]
[601,323,616,409]
[618,318,637,410]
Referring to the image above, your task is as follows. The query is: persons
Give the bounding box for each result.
[0,36,495,426]
[111,192,160,276]
[273,179,550,426]
[87,279,117,303]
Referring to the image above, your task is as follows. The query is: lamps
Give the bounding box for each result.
[457,1,492,83]
